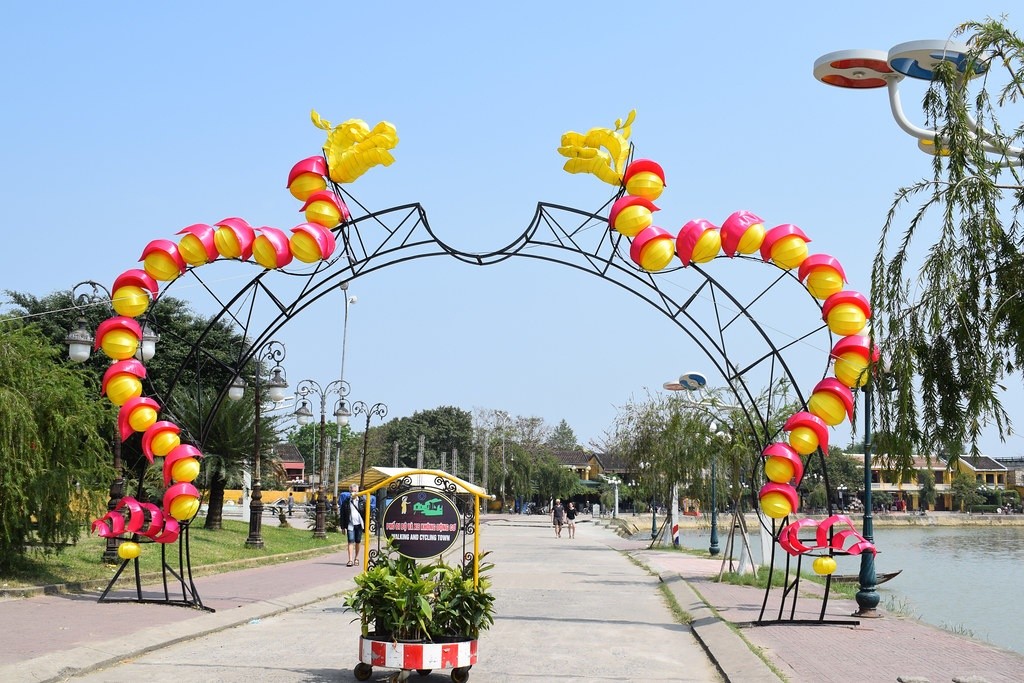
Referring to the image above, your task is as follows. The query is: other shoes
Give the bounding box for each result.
[569,536,572,539]
[354,559,359,566]
[347,561,353,566]
[558,532,561,538]
[572,535,575,539]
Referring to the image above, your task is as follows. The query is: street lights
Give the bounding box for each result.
[333,281,357,518]
[837,484,848,516]
[849,346,899,619]
[294,378,388,538]
[229,338,288,549]
[690,422,733,556]
[639,452,657,538]
[495,411,514,516]
[628,478,640,515]
[62,280,161,565]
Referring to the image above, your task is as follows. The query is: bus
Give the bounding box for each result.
[198,483,312,505]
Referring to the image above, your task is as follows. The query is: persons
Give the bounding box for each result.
[586,500,594,515]
[340,483,365,567]
[551,499,577,539]
[285,493,295,511]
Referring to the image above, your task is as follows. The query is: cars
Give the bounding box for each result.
[518,507,532,515]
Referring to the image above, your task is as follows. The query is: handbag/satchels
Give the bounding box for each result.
[360,513,365,533]
